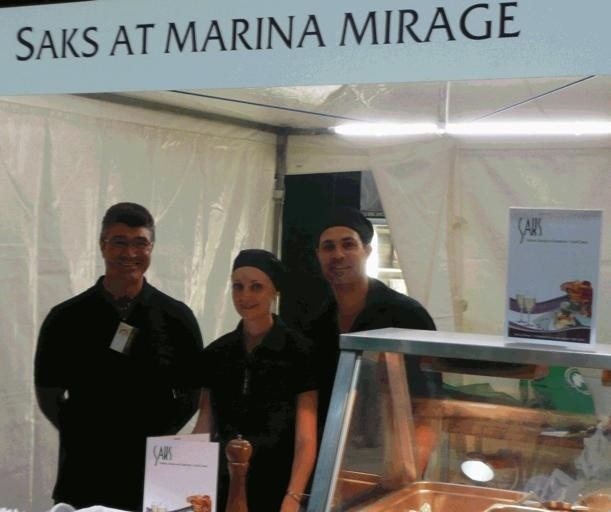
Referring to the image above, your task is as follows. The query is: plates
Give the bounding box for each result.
[308,326,611,512]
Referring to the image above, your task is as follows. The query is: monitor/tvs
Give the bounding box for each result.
[144,431,223,512]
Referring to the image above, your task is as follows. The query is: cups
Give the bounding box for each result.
[102,235,154,248]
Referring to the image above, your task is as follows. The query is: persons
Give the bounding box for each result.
[187,249,321,512]
[33,202,205,512]
[291,204,444,511]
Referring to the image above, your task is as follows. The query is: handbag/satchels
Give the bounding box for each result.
[287,489,306,506]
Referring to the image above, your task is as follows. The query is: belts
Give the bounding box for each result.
[516,291,536,327]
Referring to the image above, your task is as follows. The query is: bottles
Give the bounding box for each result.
[231,248,285,293]
[318,205,376,246]
